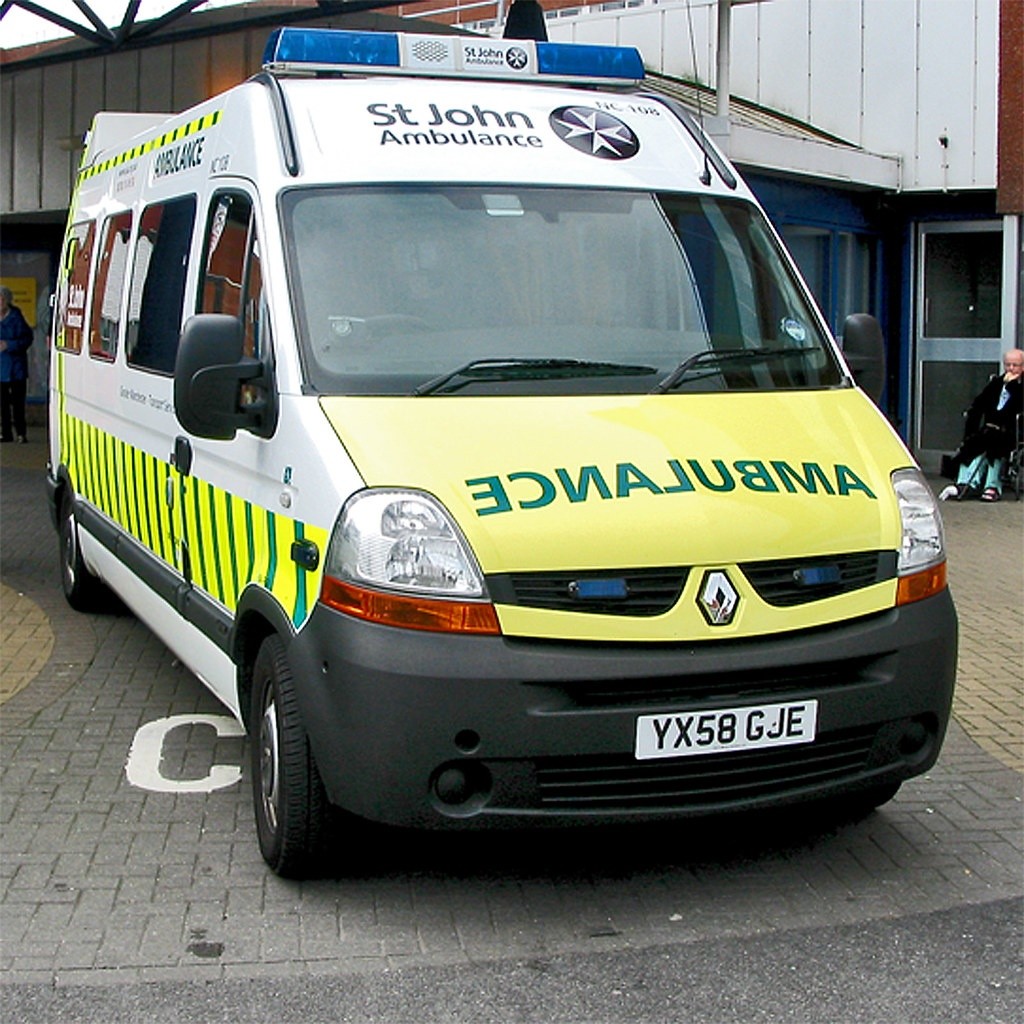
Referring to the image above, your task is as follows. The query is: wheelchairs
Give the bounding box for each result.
[963,374,1024,499]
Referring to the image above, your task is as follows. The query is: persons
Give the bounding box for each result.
[0,286,35,445]
[945,348,1024,502]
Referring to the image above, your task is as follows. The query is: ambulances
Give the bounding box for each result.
[42,26,961,878]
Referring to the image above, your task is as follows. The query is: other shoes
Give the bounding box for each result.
[951,485,978,499]
[980,488,1002,501]
[0,436,13,442]
[18,436,27,443]
